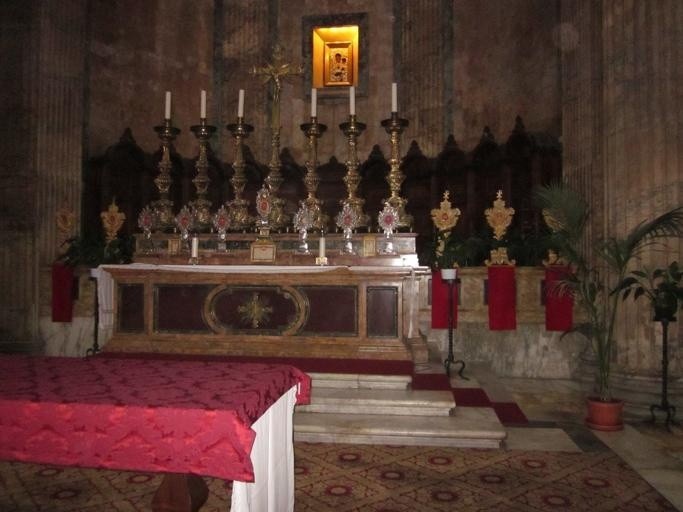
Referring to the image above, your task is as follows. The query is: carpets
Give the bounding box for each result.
[0,421,680,512]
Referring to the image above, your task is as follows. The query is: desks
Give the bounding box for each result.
[1,352,315,512]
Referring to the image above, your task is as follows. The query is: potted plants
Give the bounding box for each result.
[603,258,682,319]
[534,177,683,433]
[435,234,487,282]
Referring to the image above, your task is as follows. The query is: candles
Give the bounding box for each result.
[391,82,398,112]
[316,235,326,259]
[164,91,171,120]
[186,237,200,260]
[349,86,356,115]
[200,89,207,120]
[55,234,129,277]
[237,89,245,117]
[311,88,317,117]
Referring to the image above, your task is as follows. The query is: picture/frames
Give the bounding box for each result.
[321,39,354,88]
